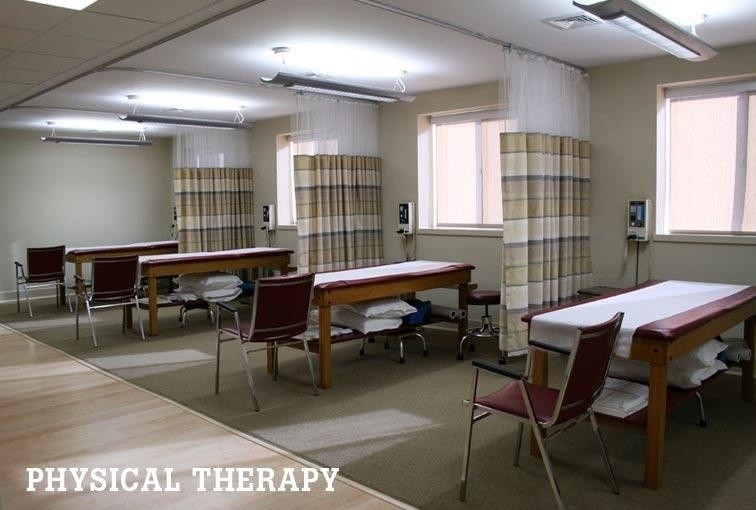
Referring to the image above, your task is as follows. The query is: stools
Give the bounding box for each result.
[458,288,510,365]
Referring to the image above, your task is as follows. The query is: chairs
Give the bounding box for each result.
[209,272,324,414]
[12,241,152,348]
[449,311,628,508]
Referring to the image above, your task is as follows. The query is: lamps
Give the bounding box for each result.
[38,119,154,153]
[569,0,724,64]
[114,93,255,131]
[256,42,419,104]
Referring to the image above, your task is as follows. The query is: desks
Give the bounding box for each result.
[266,258,477,385]
[521,274,756,489]
[63,237,295,337]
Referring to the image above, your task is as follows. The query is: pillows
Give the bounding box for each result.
[609,338,732,389]
[310,298,421,338]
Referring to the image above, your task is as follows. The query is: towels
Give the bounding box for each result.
[526,280,753,358]
[593,375,652,421]
[310,259,470,298]
